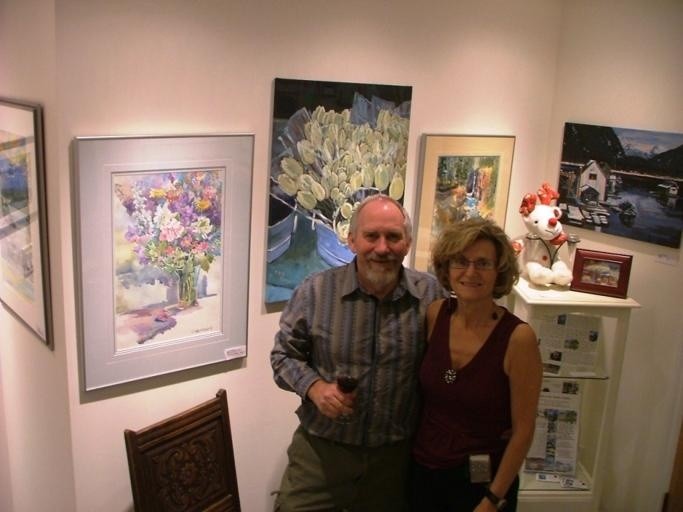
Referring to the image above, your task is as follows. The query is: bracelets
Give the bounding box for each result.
[483,486,507,510]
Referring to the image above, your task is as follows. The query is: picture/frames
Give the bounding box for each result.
[75,133,256,394]
[0,97,56,352]
[570,248,633,299]
[410,133,515,298]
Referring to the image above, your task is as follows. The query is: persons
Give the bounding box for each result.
[410,215,541,511]
[269,194,452,512]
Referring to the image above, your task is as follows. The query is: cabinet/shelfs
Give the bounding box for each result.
[495,273,644,512]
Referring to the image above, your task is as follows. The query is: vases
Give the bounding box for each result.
[177,267,197,306]
[267,196,298,263]
[315,222,358,269]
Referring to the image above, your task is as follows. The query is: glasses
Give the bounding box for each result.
[446,255,500,272]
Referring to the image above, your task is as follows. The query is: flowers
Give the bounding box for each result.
[269,92,410,247]
[116,169,223,304]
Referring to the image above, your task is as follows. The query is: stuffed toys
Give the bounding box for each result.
[510,182,573,287]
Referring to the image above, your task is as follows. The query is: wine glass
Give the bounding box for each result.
[334,366,359,425]
